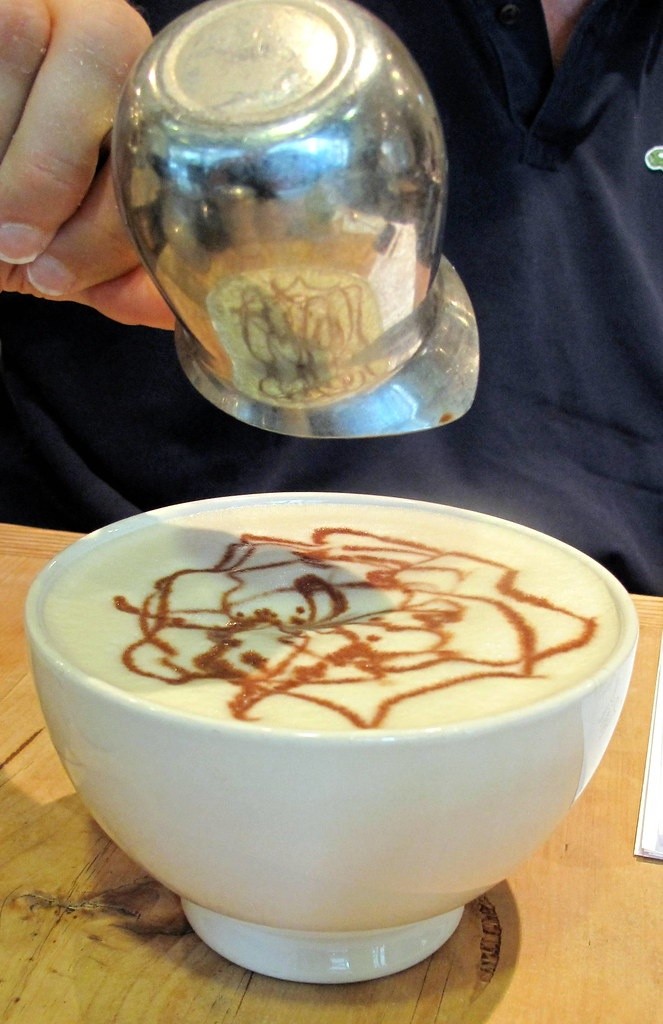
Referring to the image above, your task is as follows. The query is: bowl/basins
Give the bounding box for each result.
[24,490,642,985]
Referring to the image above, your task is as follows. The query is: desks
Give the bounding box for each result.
[0,518,663,1020]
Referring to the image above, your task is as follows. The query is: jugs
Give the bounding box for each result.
[95,0,481,441]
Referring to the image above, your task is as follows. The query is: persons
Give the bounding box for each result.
[0,0,662,598]
